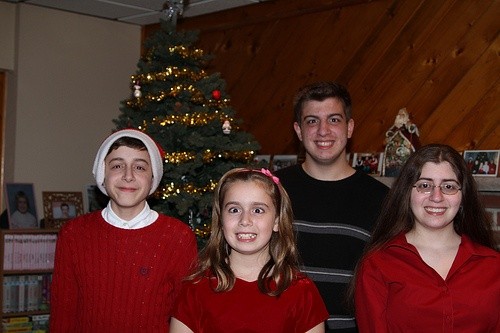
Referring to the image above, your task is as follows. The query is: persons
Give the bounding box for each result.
[167,163,330,333]
[11,191,36,227]
[47,128,199,333]
[467,155,496,175]
[59,203,72,218]
[266,81,391,333]
[347,145,500,333]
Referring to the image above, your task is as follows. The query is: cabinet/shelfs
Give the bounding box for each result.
[0,227,63,333]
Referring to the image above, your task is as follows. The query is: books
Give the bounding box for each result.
[1,274,53,313]
[3,233,57,271]
[1,315,49,333]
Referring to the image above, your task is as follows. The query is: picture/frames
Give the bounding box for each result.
[249,153,272,174]
[39,189,85,230]
[86,183,110,216]
[4,179,42,229]
[352,153,384,177]
[463,149,500,178]
[271,155,303,174]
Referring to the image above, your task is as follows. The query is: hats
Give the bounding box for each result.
[92,129,165,196]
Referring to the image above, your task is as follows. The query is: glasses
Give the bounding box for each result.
[412,182,463,194]
